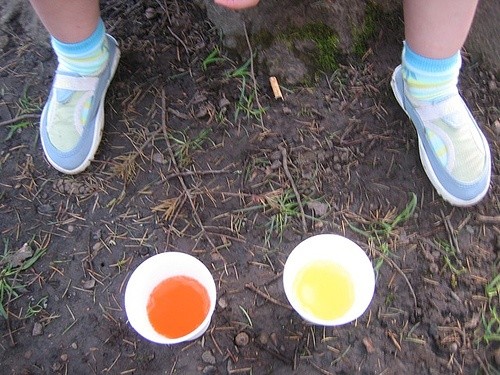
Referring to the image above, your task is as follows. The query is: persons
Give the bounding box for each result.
[26,0,491,208]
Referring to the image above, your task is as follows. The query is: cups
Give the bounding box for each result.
[125,252,217,345]
[282,234,375,326]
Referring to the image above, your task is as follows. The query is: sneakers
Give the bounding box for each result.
[391,64,491,206]
[39,33,120,175]
